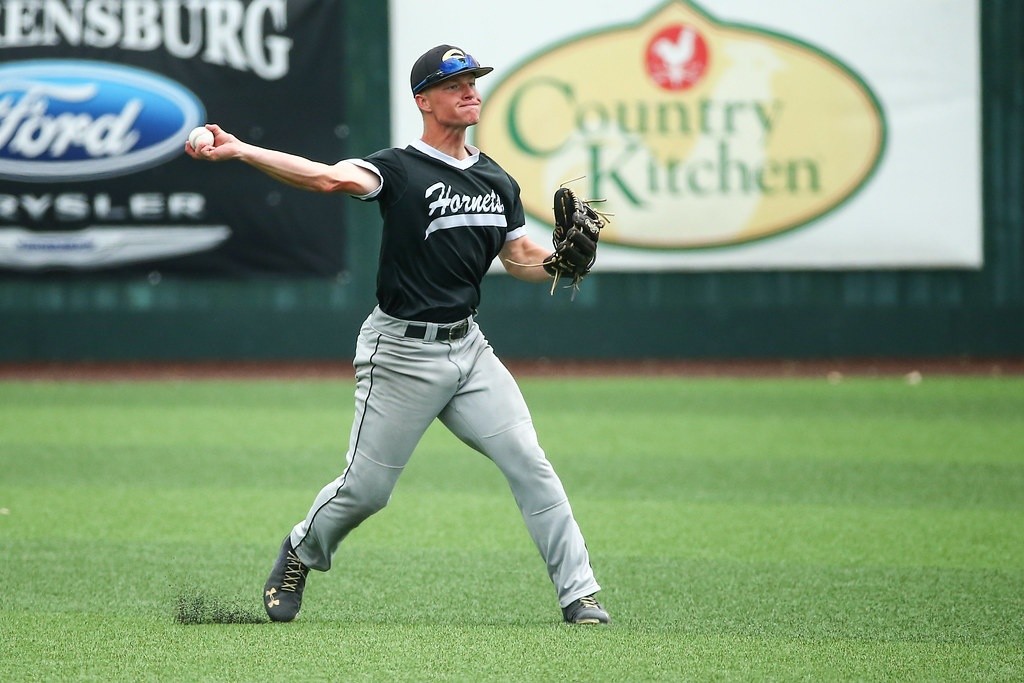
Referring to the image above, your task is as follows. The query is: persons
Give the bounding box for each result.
[185,45,609,624]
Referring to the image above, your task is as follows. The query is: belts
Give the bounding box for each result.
[404,320,468,342]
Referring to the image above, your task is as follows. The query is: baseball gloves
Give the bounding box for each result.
[503,174,616,303]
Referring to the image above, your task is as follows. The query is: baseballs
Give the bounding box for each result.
[188,126,215,151]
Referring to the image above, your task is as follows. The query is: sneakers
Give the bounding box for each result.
[262,534,309,623]
[564,595,608,625]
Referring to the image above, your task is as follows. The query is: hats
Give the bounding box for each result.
[410,44,493,98]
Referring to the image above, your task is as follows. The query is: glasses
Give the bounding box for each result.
[412,54,480,92]
